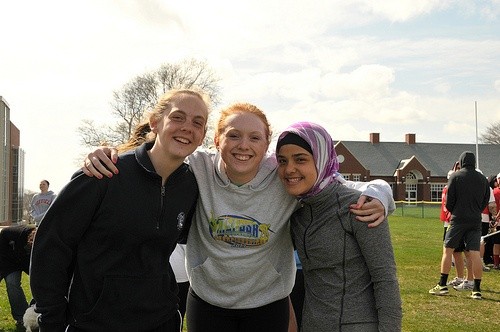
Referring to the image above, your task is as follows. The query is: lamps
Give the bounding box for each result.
[426,177,430,183]
[401,177,405,183]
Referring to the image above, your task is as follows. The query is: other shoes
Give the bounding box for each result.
[493,254,500,269]
[17,319,26,332]
[482,264,491,271]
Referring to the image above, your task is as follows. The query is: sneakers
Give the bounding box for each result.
[448,276,464,286]
[471,290,482,299]
[429,284,449,294]
[453,280,474,290]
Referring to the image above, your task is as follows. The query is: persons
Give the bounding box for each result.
[288,250,307,332]
[31,179,56,227]
[275,121,403,332]
[169,243,192,332]
[81,101,397,332]
[428,152,500,298]
[0,224,41,332]
[30,88,211,332]
